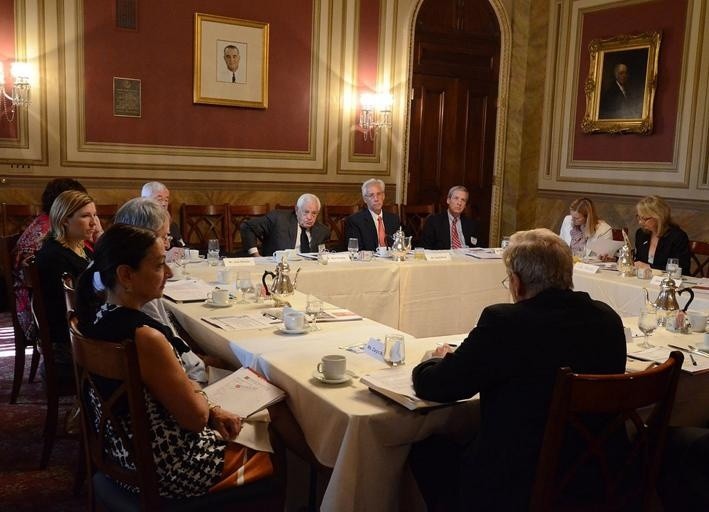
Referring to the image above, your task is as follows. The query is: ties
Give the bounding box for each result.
[377,216,385,247]
[299,226,310,253]
[452,216,461,249]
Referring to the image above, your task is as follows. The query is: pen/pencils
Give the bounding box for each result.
[690,355,697,366]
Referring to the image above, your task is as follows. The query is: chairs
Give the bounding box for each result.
[1,199,709,511]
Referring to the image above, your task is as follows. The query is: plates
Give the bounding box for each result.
[312,371,353,385]
[286,257,302,261]
[205,299,236,308]
[279,327,306,334]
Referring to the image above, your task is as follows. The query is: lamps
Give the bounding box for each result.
[353,80,394,143]
[0,60,40,118]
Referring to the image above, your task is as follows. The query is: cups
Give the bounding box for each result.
[284,248,297,258]
[317,252,328,265]
[316,354,347,379]
[501,239,509,249]
[655,308,709,334]
[635,261,650,278]
[206,289,229,303]
[216,267,234,284]
[282,310,305,329]
[167,245,199,282]
[361,247,426,262]
[253,282,271,304]
[703,332,709,349]
[666,258,682,280]
[383,333,406,367]
[207,239,220,267]
[272,251,289,263]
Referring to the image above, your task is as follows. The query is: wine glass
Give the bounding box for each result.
[346,238,360,264]
[307,300,321,333]
[235,270,254,305]
[636,306,658,349]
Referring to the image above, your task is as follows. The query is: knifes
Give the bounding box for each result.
[666,343,709,358]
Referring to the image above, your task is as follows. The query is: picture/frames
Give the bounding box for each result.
[111,1,139,34]
[112,75,144,119]
[577,27,660,138]
[190,7,271,109]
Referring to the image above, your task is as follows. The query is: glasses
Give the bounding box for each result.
[636,214,653,222]
[502,271,512,291]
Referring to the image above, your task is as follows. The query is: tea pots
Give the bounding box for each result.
[261,256,303,309]
[388,226,413,261]
[641,273,694,330]
[617,244,634,276]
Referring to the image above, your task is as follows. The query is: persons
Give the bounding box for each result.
[601,61,638,115]
[343,178,401,252]
[421,185,482,250]
[405,228,628,511]
[217,45,246,83]
[84,223,331,510]
[240,193,331,257]
[81,179,233,386]
[10,176,105,370]
[568,197,613,262]
[598,195,692,276]
